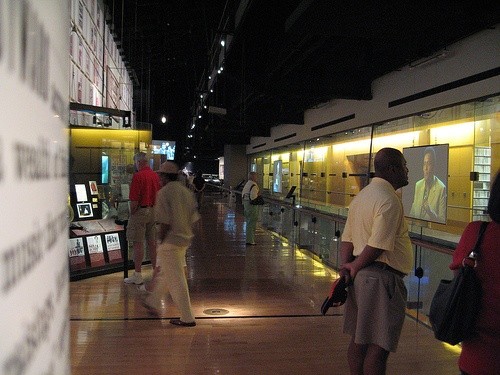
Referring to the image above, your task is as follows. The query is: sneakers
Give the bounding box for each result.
[124,271,144,285]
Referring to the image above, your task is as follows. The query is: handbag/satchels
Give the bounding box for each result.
[427,263,487,346]
[250,195,264,205]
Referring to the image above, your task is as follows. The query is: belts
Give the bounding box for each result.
[348,255,408,279]
[140,205,153,208]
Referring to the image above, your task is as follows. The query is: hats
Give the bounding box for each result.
[153,161,179,174]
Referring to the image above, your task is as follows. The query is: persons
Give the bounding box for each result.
[123,152,162,284]
[409,147,446,224]
[145,161,200,327]
[242,171,259,244]
[178,169,205,210]
[449,166,500,375]
[339,148,412,375]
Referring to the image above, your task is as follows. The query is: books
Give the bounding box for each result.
[473,199,488,205]
[474,191,490,197]
[474,207,488,214]
[475,157,491,164]
[479,174,490,180]
[474,182,490,189]
[475,148,491,155]
[473,216,488,221]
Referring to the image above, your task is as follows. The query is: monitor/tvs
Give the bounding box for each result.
[151,140,176,160]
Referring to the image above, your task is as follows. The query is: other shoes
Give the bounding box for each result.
[170,317,196,327]
[138,298,161,312]
[246,242,256,246]
